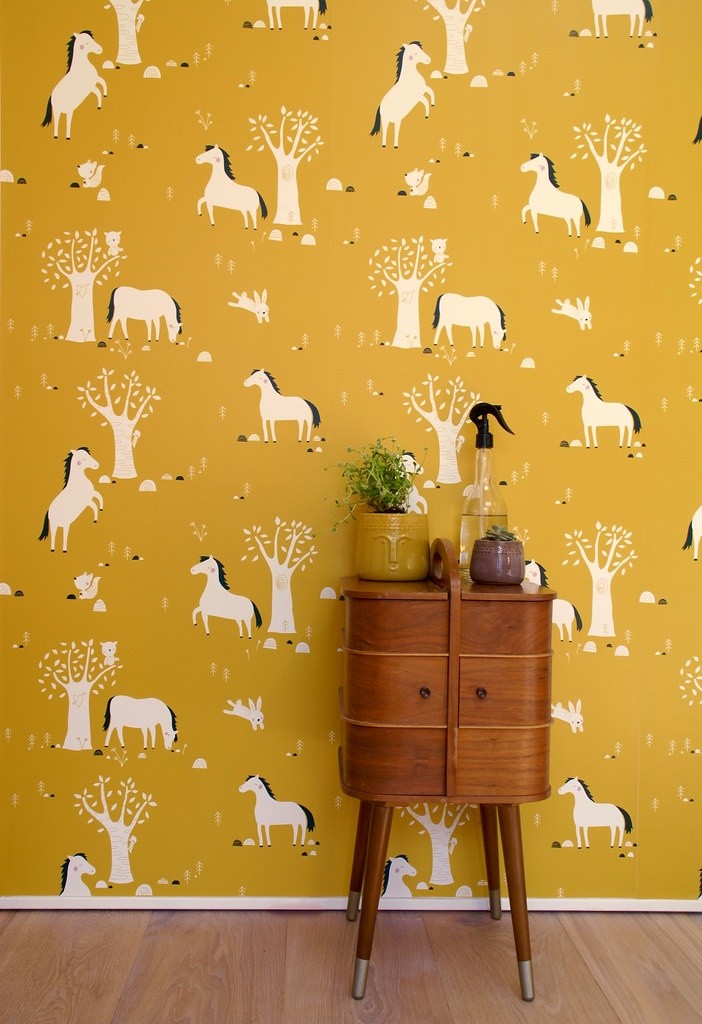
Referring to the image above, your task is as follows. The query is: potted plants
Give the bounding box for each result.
[337,436,430,582]
[469,525,526,587]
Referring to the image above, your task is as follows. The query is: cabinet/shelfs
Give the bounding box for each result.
[339,538,557,1003]
